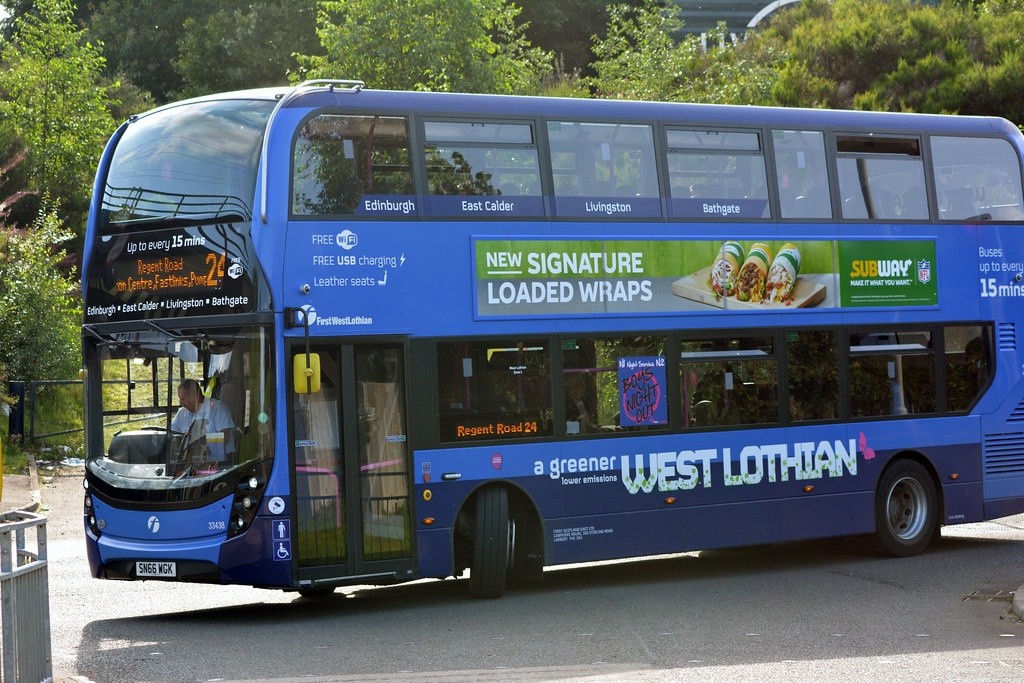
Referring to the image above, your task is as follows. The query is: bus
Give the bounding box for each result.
[75,76,1024,601]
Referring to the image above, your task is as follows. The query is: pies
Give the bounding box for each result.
[707,240,802,304]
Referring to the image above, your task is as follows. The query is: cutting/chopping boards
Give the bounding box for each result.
[672,264,826,310]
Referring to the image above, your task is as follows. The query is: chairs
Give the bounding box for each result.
[500,180,979,220]
[694,400,716,426]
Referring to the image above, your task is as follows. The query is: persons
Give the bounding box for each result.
[945,337,987,404]
[170,379,236,466]
